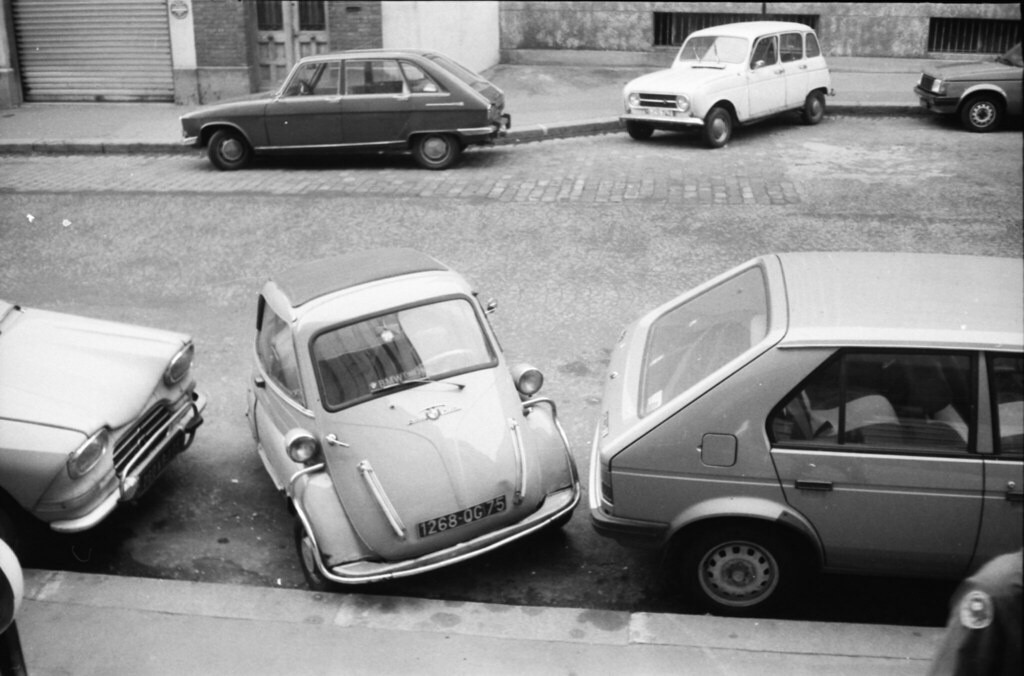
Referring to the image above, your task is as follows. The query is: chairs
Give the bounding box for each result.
[795,364,1023,461]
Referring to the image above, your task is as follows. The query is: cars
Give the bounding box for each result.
[181,47,511,169]
[617,21,835,147]
[580,252,1024,619]
[245,245,580,596]
[0,296,209,568]
[913,41,1023,131]
